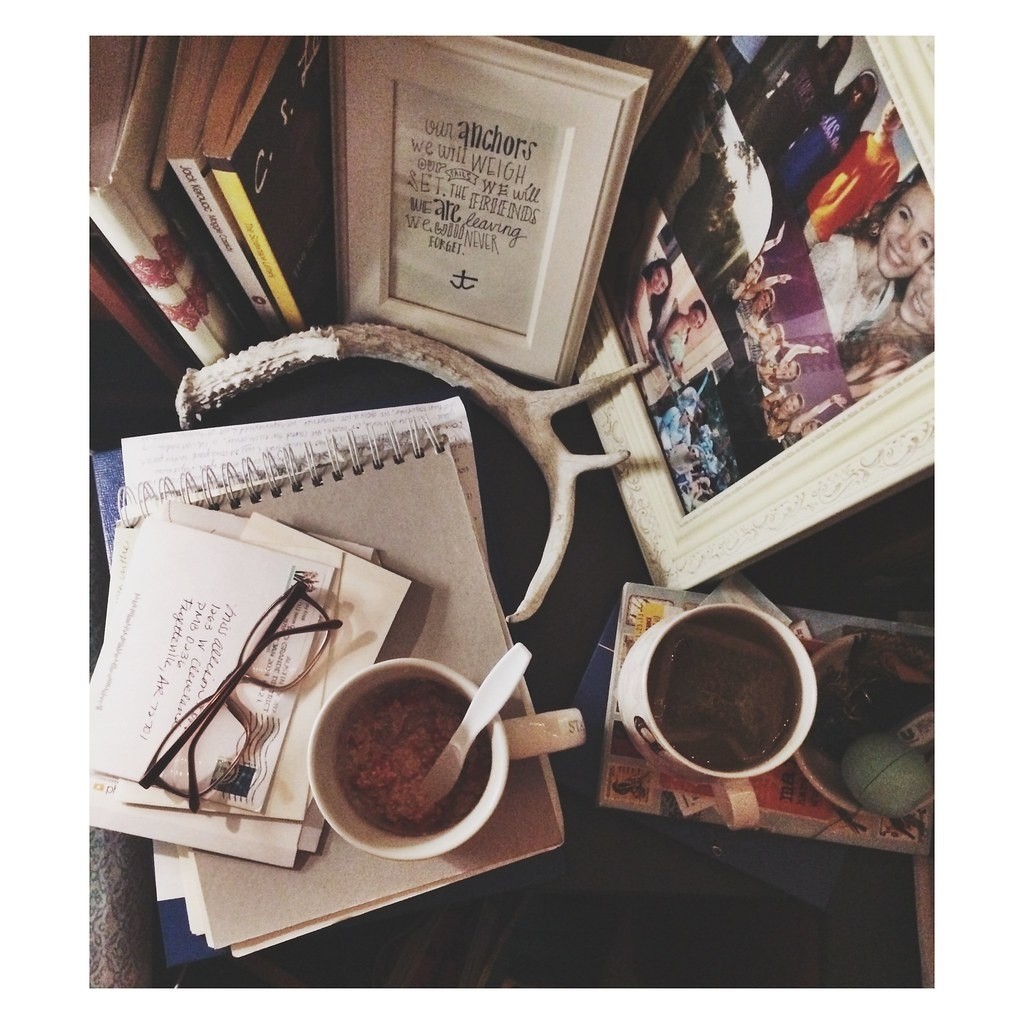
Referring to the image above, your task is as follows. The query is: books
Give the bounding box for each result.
[91,420,564,966]
[90,35,340,397]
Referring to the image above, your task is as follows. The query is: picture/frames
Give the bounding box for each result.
[578,36,935,593]
[323,36,655,387]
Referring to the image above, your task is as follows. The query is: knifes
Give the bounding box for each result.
[421,643,535,801]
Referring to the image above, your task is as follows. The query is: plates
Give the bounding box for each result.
[794,631,935,817]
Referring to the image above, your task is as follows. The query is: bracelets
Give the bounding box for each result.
[670,356,675,362]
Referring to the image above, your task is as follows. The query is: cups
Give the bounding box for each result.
[307,657,588,860]
[619,603,818,831]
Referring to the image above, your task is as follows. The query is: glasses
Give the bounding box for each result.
[138,581,343,814]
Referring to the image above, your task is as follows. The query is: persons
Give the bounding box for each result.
[812,165,934,402]
[720,35,902,274]
[652,385,736,506]
[711,253,847,449]
[633,257,706,381]
